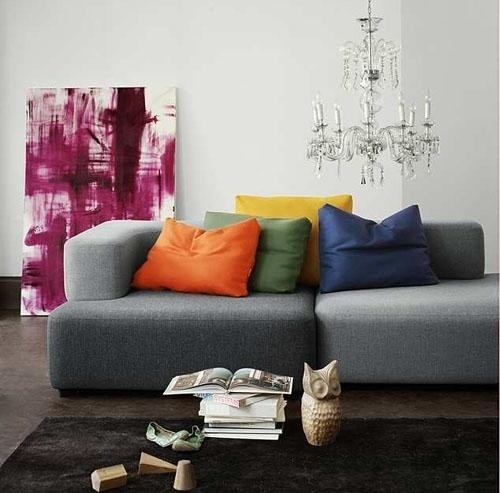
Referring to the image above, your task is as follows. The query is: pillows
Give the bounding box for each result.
[130,217,261,298]
[317,203,440,293]
[204,211,312,294]
[234,194,353,287]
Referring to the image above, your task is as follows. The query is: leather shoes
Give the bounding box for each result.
[172,426,204,451]
[146,422,188,447]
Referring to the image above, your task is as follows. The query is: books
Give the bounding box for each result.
[193,393,289,441]
[162,367,295,395]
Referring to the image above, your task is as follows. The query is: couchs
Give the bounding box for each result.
[45,214,500,399]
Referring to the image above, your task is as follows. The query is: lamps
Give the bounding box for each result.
[305,0,444,194]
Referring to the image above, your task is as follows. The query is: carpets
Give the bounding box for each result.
[1,409,499,493]
